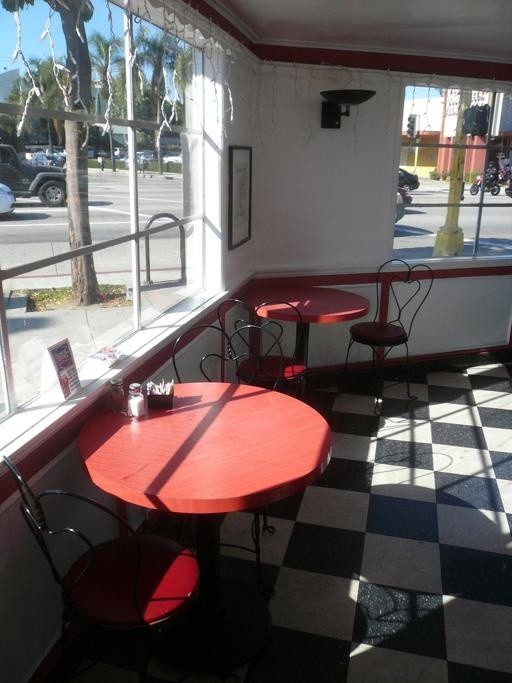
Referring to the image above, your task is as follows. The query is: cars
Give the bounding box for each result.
[121,151,183,169]
[395,168,420,223]
[0,182,14,215]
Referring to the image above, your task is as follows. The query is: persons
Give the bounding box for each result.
[480,160,498,191]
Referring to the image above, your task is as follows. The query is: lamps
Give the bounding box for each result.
[320,90,377,129]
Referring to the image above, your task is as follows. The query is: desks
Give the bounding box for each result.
[79,382,333,608]
[244,285,370,367]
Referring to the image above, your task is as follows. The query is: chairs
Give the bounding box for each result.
[3,455,245,683]
[344,259,435,417]
[173,324,284,568]
[217,299,306,399]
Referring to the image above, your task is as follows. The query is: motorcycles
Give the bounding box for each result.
[470,171,500,196]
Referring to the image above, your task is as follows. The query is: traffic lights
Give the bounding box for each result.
[407,114,416,138]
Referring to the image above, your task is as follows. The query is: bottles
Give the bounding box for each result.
[106,377,125,414]
[126,382,145,418]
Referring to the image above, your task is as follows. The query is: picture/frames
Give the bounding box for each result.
[227,145,252,250]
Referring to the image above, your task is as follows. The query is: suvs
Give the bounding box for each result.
[0,144,67,207]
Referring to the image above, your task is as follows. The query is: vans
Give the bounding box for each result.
[114,146,124,155]
